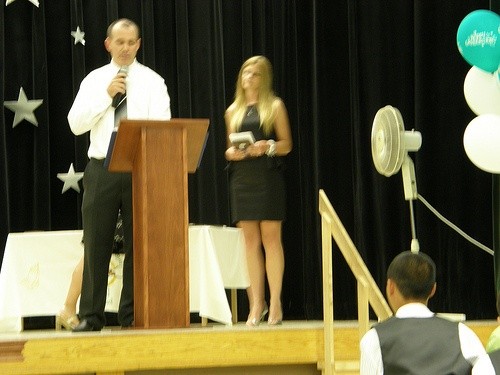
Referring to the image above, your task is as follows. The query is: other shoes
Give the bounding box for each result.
[73,319,102,331]
[121,323,131,329]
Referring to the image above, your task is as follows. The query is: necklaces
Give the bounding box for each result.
[250,107,253,112]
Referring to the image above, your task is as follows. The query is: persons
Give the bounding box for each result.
[359,251,496,375]
[56,18,171,331]
[224,56,292,326]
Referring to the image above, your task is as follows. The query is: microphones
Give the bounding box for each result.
[112,65,127,108]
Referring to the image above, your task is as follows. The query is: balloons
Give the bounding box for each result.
[457,10,500,175]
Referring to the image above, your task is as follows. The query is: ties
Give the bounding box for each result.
[115,68,127,127]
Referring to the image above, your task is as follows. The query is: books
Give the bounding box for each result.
[229,132,255,150]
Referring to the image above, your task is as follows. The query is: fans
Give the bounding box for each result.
[371,105,467,322]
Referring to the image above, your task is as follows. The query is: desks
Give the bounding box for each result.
[0,225,250,331]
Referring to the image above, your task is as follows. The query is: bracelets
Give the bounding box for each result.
[266,139,275,157]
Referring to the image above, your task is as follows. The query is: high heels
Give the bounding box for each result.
[55,311,80,330]
[245,300,268,326]
[268,306,282,325]
